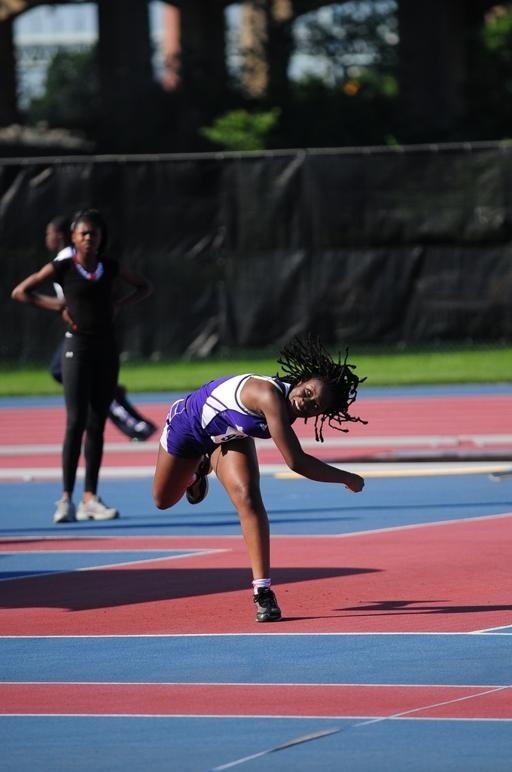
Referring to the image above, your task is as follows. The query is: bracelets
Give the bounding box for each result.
[58,304,66,317]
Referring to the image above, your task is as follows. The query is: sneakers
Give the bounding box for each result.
[251,585,282,623]
[186,455,214,505]
[76,495,118,522]
[53,498,76,524]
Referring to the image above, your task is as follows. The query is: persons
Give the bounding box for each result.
[45,212,161,442]
[12,208,118,523]
[151,333,367,620]
